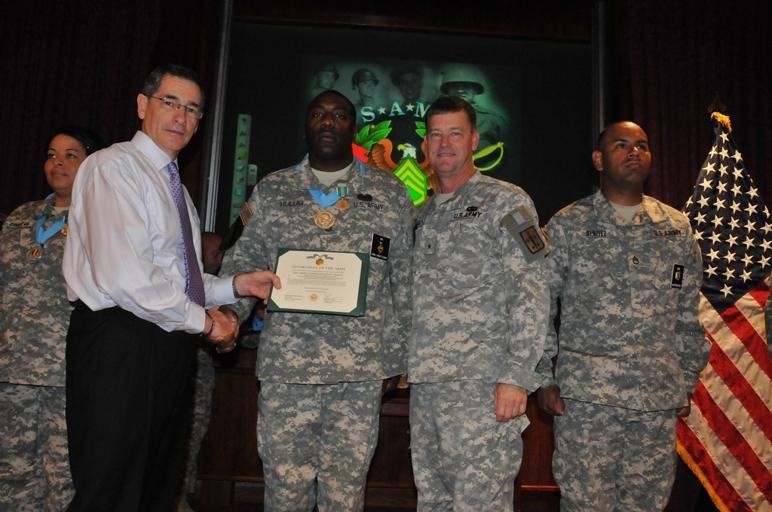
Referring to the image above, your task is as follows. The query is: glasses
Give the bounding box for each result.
[145,94,204,120]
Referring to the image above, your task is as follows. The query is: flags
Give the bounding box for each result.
[676,125,772,512]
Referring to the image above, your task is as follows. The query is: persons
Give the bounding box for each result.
[402,93,553,510]
[63,67,281,510]
[2,126,97,510]
[536,121,710,512]
[175,232,223,510]
[214,90,418,510]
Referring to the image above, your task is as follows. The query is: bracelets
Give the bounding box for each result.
[232,274,241,298]
[205,311,214,336]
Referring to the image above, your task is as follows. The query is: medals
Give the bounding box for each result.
[315,211,334,229]
[27,245,42,262]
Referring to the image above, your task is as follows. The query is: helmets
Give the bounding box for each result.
[352,69,379,84]
[313,62,339,80]
[440,63,485,94]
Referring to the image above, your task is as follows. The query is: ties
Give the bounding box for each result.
[167,161,205,307]
